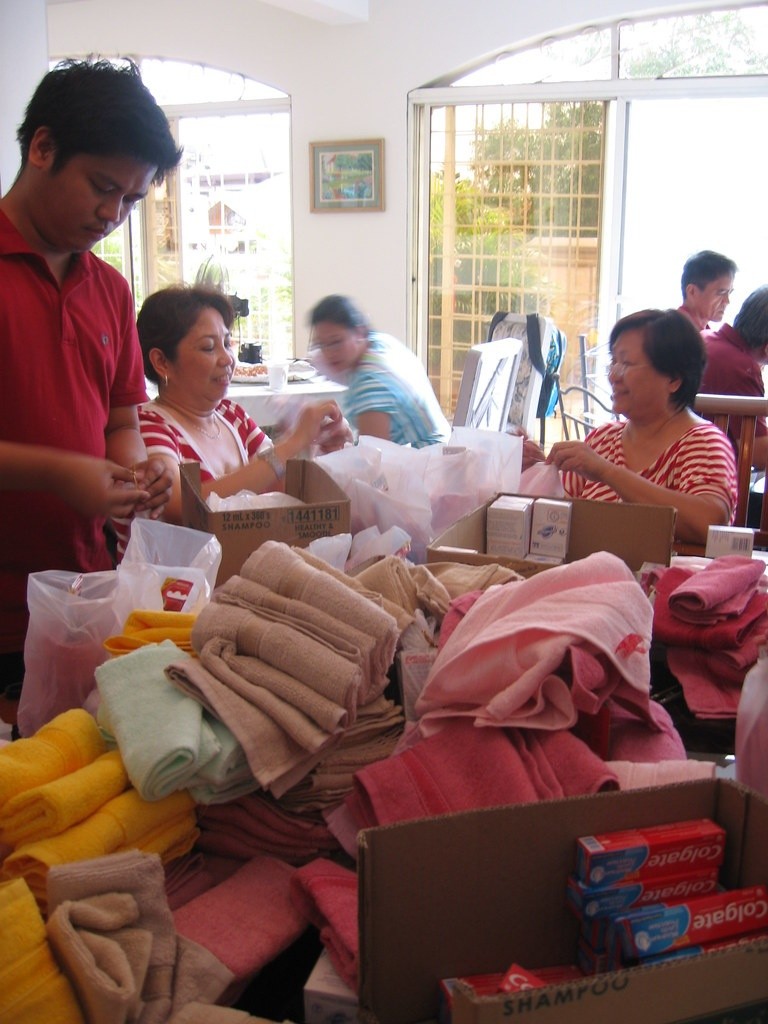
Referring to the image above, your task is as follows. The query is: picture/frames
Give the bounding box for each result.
[309,138,385,213]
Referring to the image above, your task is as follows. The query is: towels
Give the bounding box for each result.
[0,537,767,1024]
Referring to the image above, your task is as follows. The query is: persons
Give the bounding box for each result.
[692,282,768,471]
[307,291,451,449]
[0,51,182,735]
[518,307,739,546]
[677,250,740,331]
[107,282,356,568]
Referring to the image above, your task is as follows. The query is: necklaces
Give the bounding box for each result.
[154,396,221,439]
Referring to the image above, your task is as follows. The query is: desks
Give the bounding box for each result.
[142,374,348,446]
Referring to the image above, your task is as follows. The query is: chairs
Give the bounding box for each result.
[450,337,524,434]
[554,373,620,443]
[692,393,768,552]
[489,311,555,439]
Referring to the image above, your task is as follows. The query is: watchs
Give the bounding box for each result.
[257,448,285,482]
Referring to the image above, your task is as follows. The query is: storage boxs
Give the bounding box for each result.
[178,458,351,590]
[355,776,768,1024]
[425,490,678,579]
